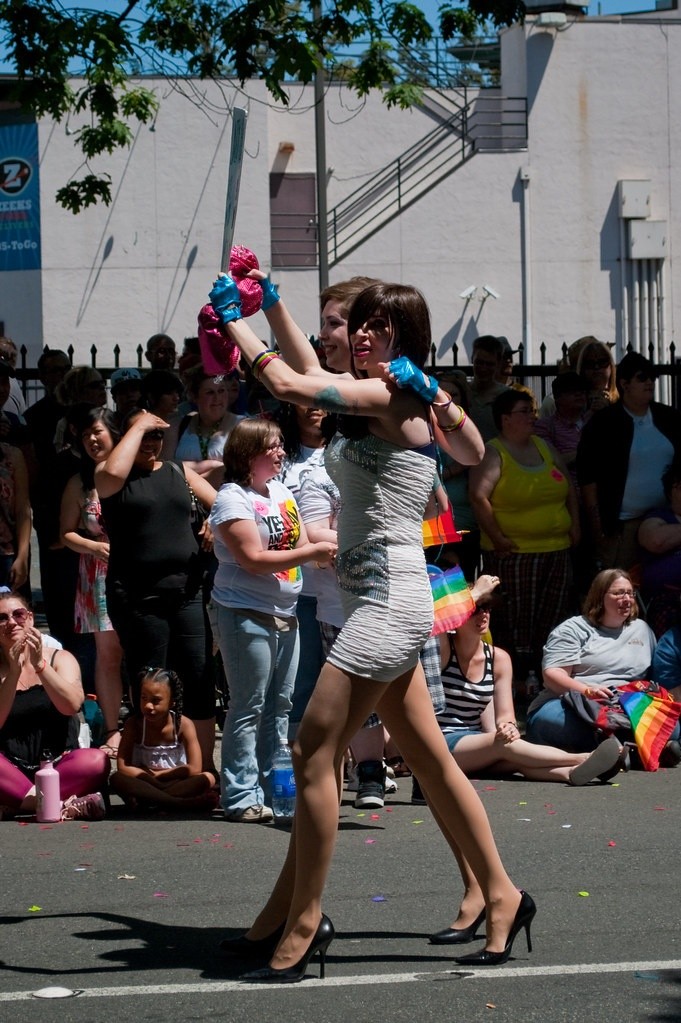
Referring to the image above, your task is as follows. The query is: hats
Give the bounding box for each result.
[0,349,16,378]
[552,373,593,396]
[111,369,143,394]
[498,337,519,356]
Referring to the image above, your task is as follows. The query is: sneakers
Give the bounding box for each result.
[411,775,427,804]
[355,761,385,808]
[59,792,106,820]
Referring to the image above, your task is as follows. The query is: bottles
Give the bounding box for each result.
[525,671,539,706]
[35,749,61,822]
[270,739,296,818]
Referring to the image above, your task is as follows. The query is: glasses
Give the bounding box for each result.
[560,387,587,393]
[471,602,492,616]
[1,608,28,625]
[607,591,636,598]
[507,409,537,416]
[149,348,179,357]
[142,429,164,441]
[583,358,609,371]
[634,372,657,383]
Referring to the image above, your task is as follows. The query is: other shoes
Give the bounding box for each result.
[230,804,274,822]
[347,760,398,792]
[563,740,680,786]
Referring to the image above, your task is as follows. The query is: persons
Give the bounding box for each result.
[198,244,537,983]
[652,621,681,739]
[208,415,340,819]
[0,586,111,821]
[438,574,631,786]
[219,260,521,967]
[528,568,681,768]
[468,389,582,683]
[432,334,681,686]
[110,666,219,821]
[0,332,242,807]
[276,400,446,809]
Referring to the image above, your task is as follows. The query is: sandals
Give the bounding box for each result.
[99,729,121,759]
[203,767,221,794]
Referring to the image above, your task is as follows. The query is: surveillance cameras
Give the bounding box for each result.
[483,285,499,300]
[460,286,477,299]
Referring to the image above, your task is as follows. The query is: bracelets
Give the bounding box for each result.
[437,406,466,433]
[433,390,452,407]
[35,659,46,673]
[316,561,327,570]
[250,348,280,378]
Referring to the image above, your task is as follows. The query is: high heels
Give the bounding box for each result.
[453,891,537,966]
[240,912,335,985]
[431,904,486,946]
[216,920,287,957]
